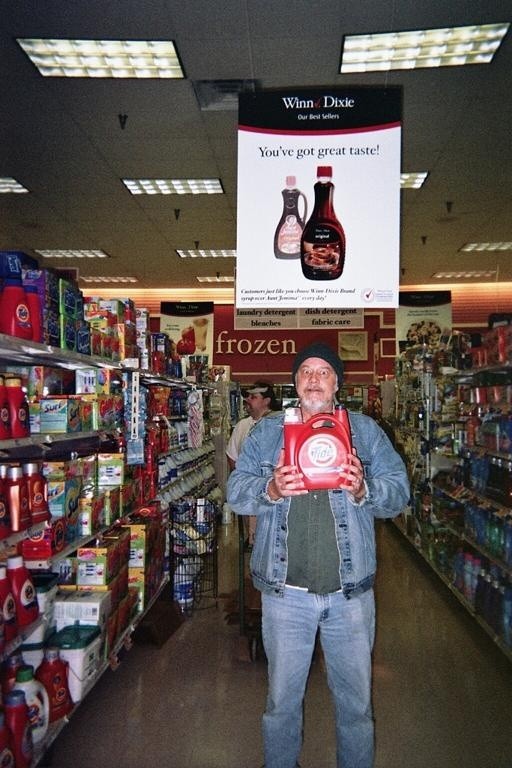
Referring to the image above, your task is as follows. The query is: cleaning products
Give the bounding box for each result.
[283,403,355,492]
[2,275,45,344]
[0,646,77,768]
[0,376,52,645]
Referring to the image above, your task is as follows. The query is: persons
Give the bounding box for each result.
[226,344,411,768]
[226,380,276,535]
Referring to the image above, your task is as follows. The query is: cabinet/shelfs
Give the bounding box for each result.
[0,329,237,766]
[377,314,512,684]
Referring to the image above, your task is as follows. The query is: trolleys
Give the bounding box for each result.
[236,513,262,668]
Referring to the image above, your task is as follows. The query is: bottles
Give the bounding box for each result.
[300,165,346,281]
[161,360,234,604]
[415,493,431,523]
[455,407,512,651]
[0,279,50,768]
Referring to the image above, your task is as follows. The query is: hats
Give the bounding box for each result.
[292,343,343,388]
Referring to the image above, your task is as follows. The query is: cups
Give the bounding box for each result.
[192,318,209,350]
[463,383,512,404]
[182,327,195,344]
